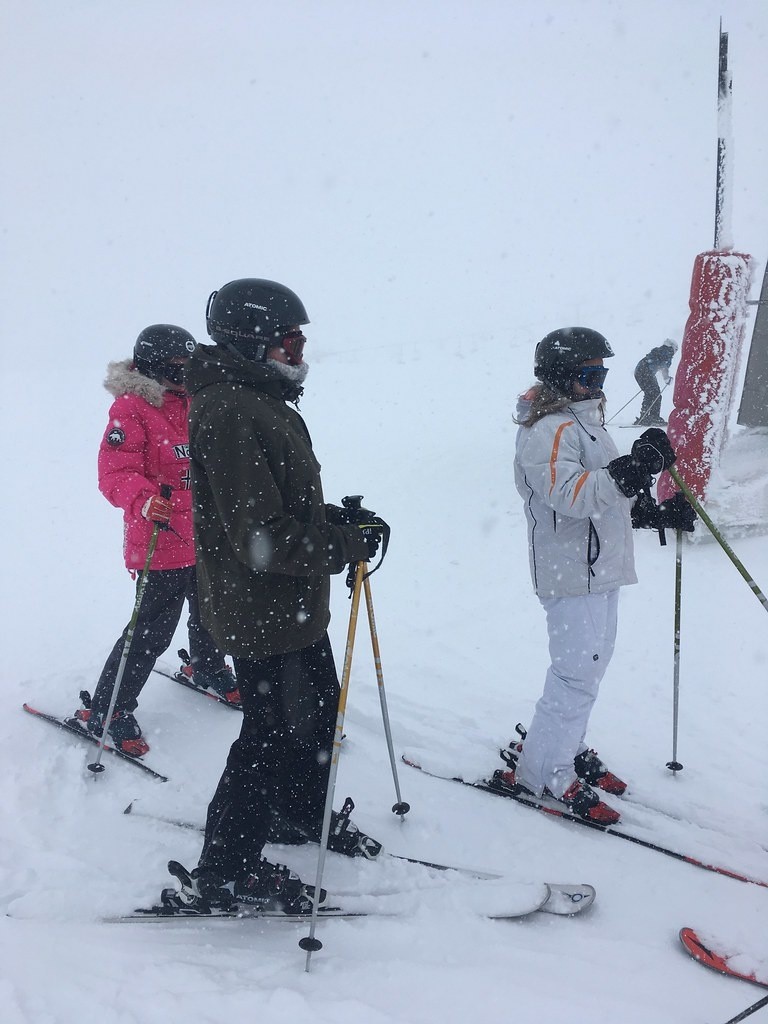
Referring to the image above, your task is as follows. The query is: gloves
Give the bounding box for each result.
[665,376,674,386]
[609,428,678,499]
[325,503,383,559]
[140,493,175,523]
[630,491,697,547]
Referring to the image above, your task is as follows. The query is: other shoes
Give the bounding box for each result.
[633,416,668,427]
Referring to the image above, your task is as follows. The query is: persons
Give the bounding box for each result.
[67,324,244,756]
[634,338,678,427]
[161,282,383,915]
[489,327,697,826]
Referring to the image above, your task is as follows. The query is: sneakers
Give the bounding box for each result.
[486,748,621,826]
[307,794,385,860]
[168,853,330,915]
[177,647,242,707]
[73,689,150,758]
[509,723,628,796]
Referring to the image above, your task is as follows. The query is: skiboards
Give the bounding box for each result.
[400,741,767,889]
[604,422,642,428]
[678,925,768,988]
[23,660,349,781]
[2,800,596,923]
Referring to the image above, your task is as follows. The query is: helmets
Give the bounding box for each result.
[663,338,679,354]
[205,278,311,363]
[133,323,197,386]
[533,326,616,396]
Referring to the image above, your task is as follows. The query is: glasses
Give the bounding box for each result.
[533,365,609,392]
[133,352,186,387]
[207,317,307,366]
